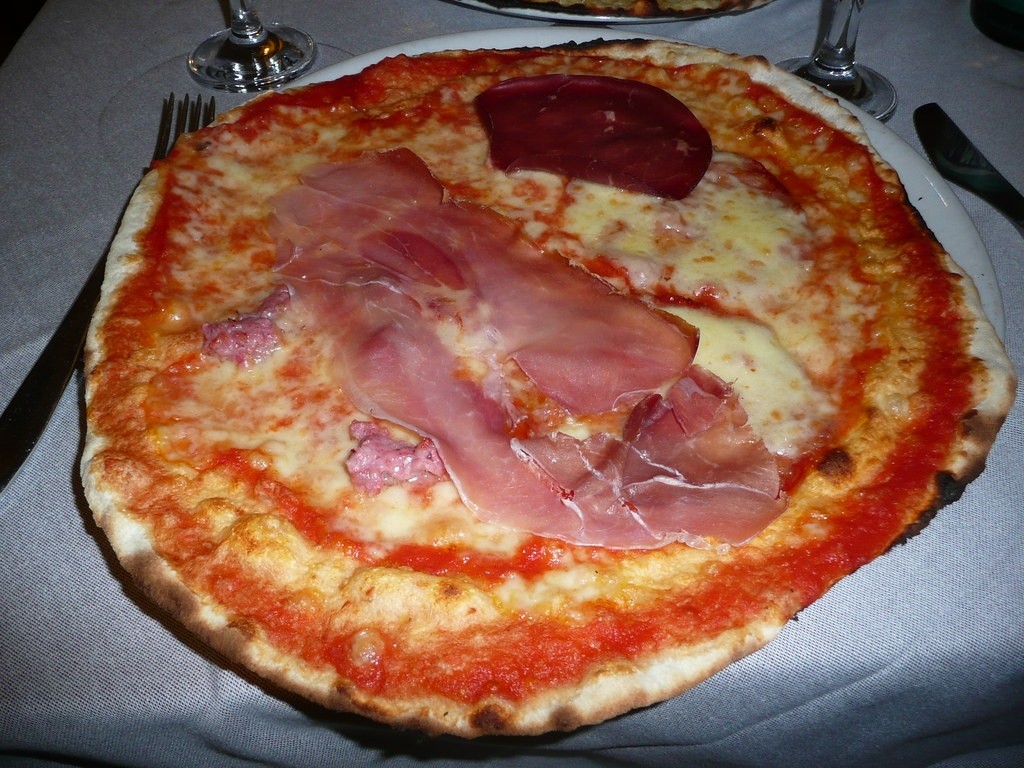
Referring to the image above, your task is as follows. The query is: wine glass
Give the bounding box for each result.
[773,0,899,121]
[189,1,316,94]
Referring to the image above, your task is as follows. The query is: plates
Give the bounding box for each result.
[449,0,777,24]
[200,25,1008,348]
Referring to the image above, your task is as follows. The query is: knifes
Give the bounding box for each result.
[913,102,1024,236]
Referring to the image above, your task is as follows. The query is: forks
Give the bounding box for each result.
[0,90,218,493]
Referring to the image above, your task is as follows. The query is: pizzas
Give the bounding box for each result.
[79,36,1016,738]
